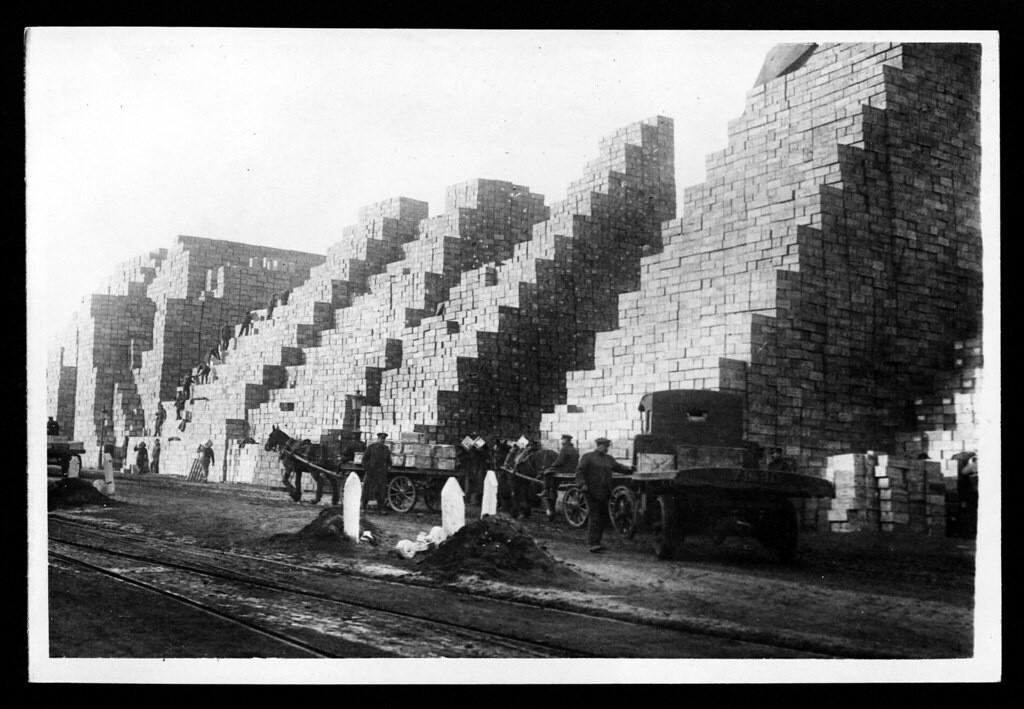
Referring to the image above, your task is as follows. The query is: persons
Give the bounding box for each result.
[237,310,254,337]
[47,417,60,436]
[359,431,392,515]
[134,442,148,476]
[536,434,579,500]
[195,359,210,384]
[197,444,214,483]
[266,293,279,320]
[575,437,636,552]
[153,402,167,436]
[336,431,367,474]
[150,439,160,473]
[220,321,231,350]
[182,371,195,400]
[173,391,186,419]
[206,345,221,365]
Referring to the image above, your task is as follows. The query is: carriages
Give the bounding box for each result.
[264,423,471,513]
[486,389,837,566]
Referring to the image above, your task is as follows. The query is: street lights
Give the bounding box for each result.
[98,405,107,470]
